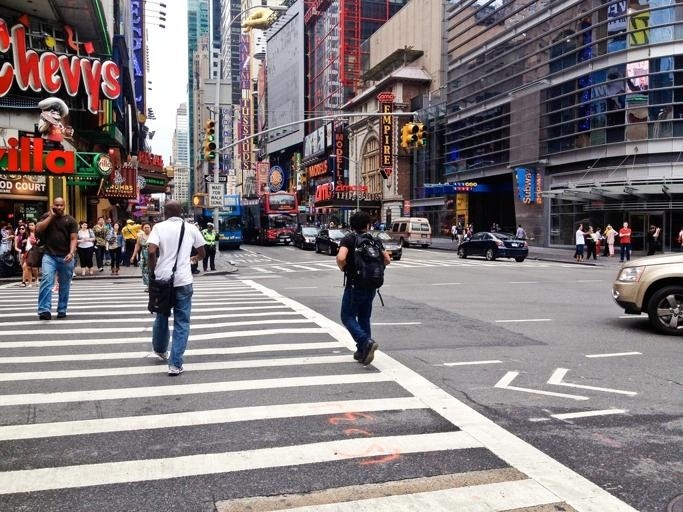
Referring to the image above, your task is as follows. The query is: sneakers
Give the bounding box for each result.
[168,364,184,374]
[57,312,66,318]
[157,352,169,360]
[40,312,51,320]
[363,339,378,365]
[354,350,363,362]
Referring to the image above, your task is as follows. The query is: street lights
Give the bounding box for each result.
[211,4,290,251]
[328,154,360,213]
[466,159,494,169]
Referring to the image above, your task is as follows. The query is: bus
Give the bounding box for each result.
[194,193,243,249]
[240,191,300,246]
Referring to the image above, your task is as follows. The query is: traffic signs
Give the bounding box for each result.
[204,174,228,184]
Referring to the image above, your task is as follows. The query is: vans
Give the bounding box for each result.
[384,217,432,249]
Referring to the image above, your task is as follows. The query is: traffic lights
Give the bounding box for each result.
[205,142,216,161]
[206,119,215,142]
[300,172,307,186]
[413,123,427,148]
[192,194,206,208]
[403,124,419,147]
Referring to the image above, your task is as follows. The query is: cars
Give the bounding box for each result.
[315,228,352,256]
[457,232,529,262]
[549,227,562,237]
[293,225,322,249]
[181,215,194,223]
[365,230,402,261]
[612,251,683,335]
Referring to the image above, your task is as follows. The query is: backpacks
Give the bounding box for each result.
[350,232,384,288]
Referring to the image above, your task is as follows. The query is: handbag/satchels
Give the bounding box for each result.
[26,244,44,266]
[148,278,176,317]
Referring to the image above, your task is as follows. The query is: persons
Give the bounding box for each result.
[514,225,527,240]
[496,224,502,233]
[451,222,473,247]
[647,225,661,257]
[26,197,78,320]
[336,211,391,366]
[491,222,497,233]
[0,216,217,293]
[575,221,632,262]
[145,199,207,376]
